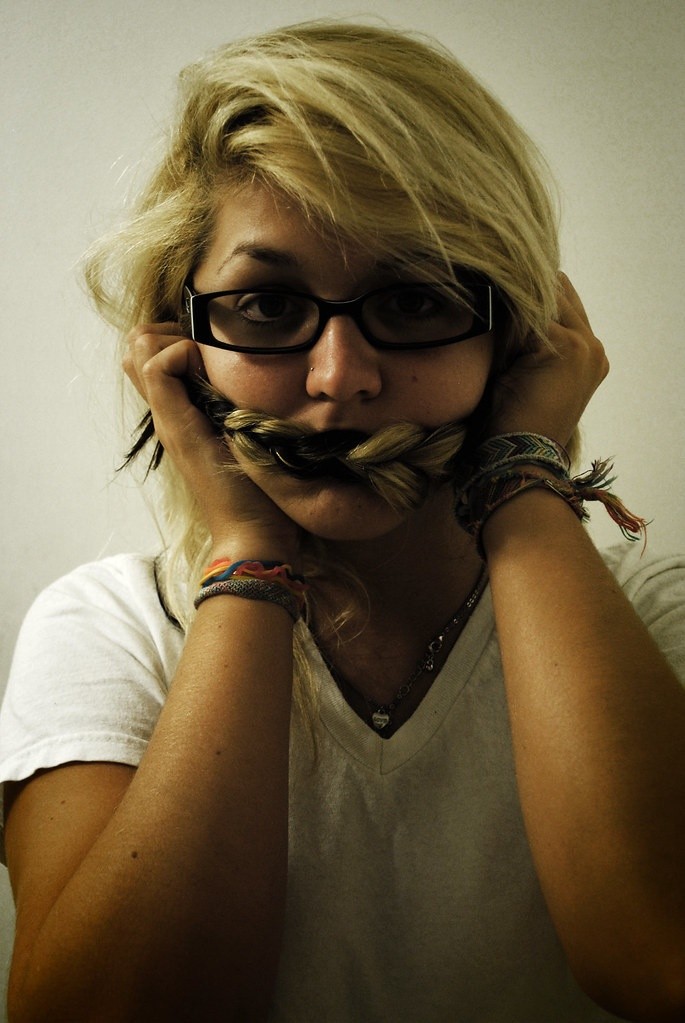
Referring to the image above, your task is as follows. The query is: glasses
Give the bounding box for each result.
[182,279,500,356]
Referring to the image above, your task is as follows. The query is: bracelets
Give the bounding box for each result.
[452,433,655,562]
[194,557,307,622]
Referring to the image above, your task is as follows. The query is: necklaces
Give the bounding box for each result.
[301,569,487,730]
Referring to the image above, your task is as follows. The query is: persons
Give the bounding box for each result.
[0,20,685,1023]
